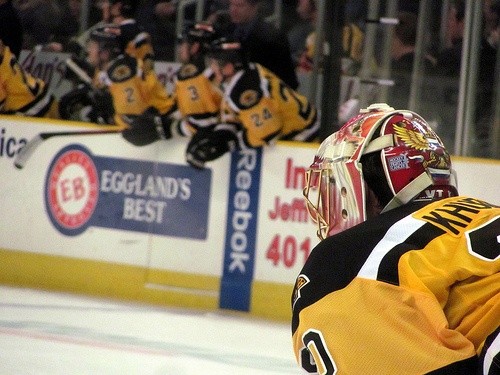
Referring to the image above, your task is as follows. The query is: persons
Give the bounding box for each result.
[151,0,211,61]
[185,36,322,171]
[481,1,499,52]
[47,0,104,50]
[288,101,500,375]
[1,0,22,61]
[293,0,366,75]
[59,24,174,127]
[116,21,225,149]
[377,10,459,156]
[69,0,154,90]
[440,0,500,159]
[1,39,59,120]
[216,0,300,90]
[14,0,60,47]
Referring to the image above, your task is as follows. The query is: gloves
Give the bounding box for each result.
[194,131,235,162]
[122,114,171,147]
[59,89,91,120]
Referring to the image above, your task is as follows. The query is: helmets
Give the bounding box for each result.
[303,102,458,241]
[177,21,216,61]
[204,37,248,91]
[90,24,123,63]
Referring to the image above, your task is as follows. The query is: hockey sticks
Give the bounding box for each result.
[14,128,123,169]
